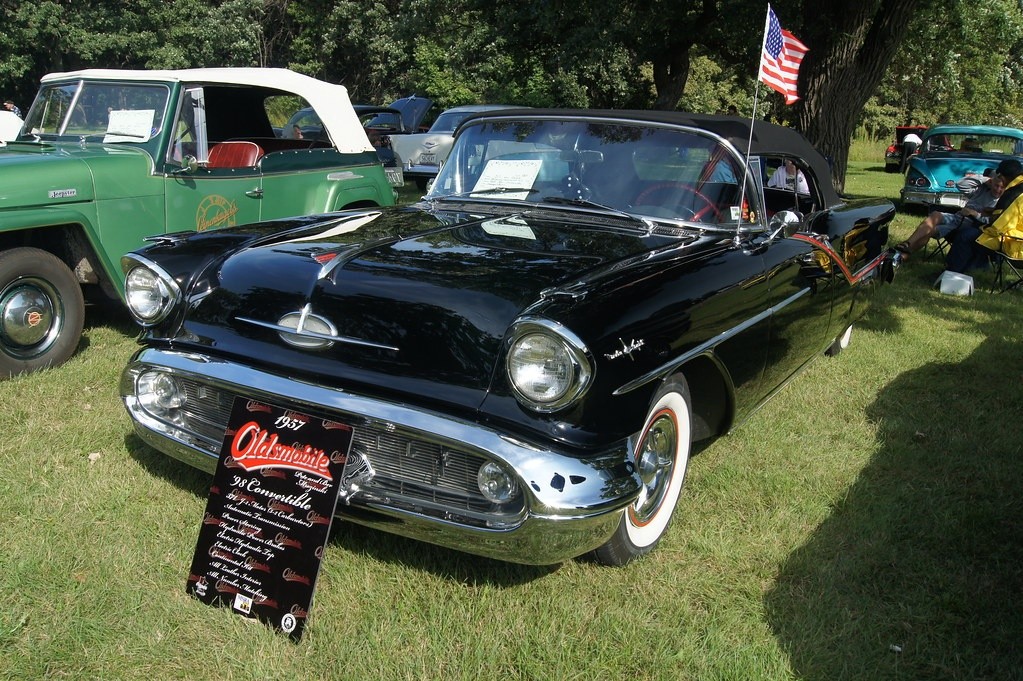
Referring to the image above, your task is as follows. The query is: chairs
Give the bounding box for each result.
[921,199,982,264]
[988,234,1023,295]
[207,140,262,166]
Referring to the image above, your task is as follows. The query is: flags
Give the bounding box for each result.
[757,1,809,107]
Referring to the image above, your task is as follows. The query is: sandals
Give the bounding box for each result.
[894,241,912,263]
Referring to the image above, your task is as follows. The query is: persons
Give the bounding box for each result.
[922,155,1023,289]
[888,177,1009,263]
[901,133,923,175]
[3,100,22,120]
[770,157,810,196]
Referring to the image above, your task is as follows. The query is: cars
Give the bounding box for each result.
[129,108,896,569]
[885,122,952,170]
[380,104,529,193]
[897,123,1022,215]
[2,66,395,378]
[273,95,432,190]
